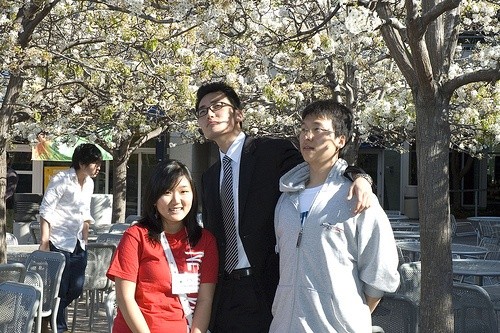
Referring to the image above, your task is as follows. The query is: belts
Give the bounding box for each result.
[220,267,254,277]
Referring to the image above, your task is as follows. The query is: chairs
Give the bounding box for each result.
[1,210,142,333]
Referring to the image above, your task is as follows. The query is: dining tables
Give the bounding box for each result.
[372,210,500,333]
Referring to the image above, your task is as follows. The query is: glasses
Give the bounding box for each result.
[299,126,335,135]
[193,103,237,117]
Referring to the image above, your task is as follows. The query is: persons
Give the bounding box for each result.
[38,142,103,333]
[270,98,401,333]
[106,158,219,333]
[6,151,19,234]
[194,81,373,333]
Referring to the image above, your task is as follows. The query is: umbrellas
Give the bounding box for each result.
[33,129,114,162]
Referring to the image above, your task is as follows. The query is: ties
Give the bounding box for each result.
[220,155,240,275]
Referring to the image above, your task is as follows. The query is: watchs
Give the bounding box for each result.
[355,168,377,188]
